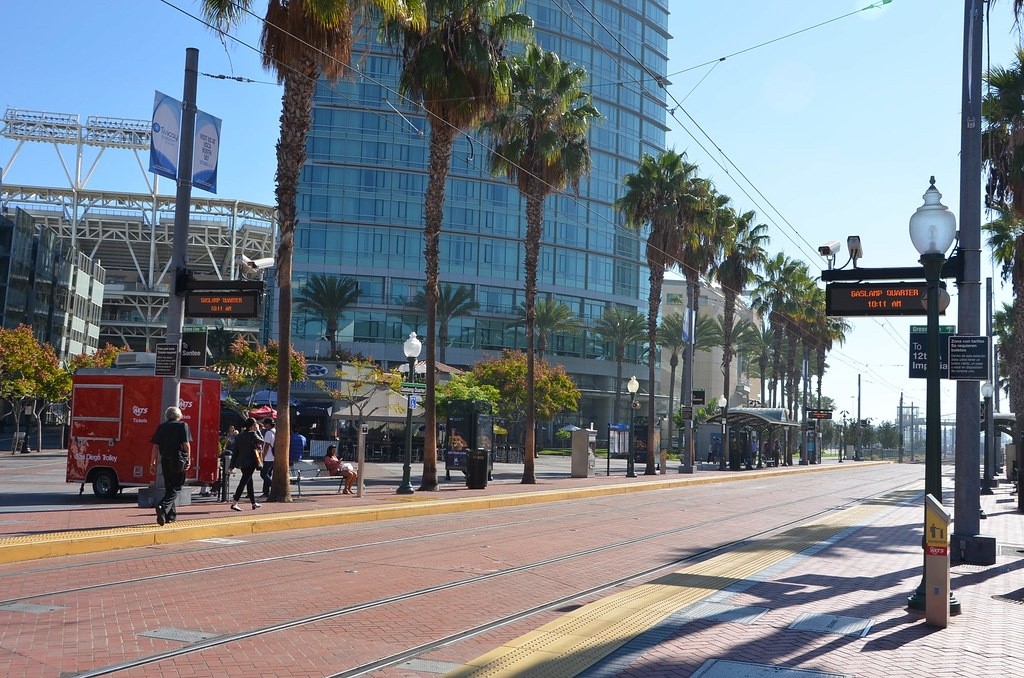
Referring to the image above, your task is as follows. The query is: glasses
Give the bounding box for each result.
[329,444,337,448]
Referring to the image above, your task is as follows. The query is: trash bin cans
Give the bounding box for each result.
[925,493,951,628]
[767,451,780,467]
[466,450,489,489]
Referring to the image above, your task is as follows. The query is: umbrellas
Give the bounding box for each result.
[562,424,580,431]
[494,423,508,434]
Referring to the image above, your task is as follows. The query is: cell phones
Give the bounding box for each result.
[340,458,343,461]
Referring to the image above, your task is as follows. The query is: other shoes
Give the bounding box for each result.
[230,504,243,511]
[156,505,165,526]
[165,517,178,524]
[349,489,356,494]
[343,490,350,494]
[252,504,261,510]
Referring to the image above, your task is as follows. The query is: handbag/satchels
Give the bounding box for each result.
[254,448,264,471]
[172,421,189,473]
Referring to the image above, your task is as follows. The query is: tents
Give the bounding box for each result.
[251,405,277,419]
[333,389,425,422]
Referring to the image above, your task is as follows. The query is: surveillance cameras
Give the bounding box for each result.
[818,240,840,256]
[848,236,863,257]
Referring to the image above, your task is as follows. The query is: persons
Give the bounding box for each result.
[325,445,357,494]
[149,406,193,526]
[706,443,716,464]
[228,417,276,512]
[798,442,802,457]
[763,439,768,456]
[752,440,757,465]
[290,423,306,477]
[775,440,782,466]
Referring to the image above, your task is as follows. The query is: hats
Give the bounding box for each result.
[245,417,257,427]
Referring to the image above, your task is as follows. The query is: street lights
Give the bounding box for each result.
[907,174,962,616]
[980,378,995,496]
[394,332,422,495]
[838,418,843,463]
[625,374,640,478]
[717,394,727,471]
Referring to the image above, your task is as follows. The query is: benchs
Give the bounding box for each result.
[289,455,358,499]
